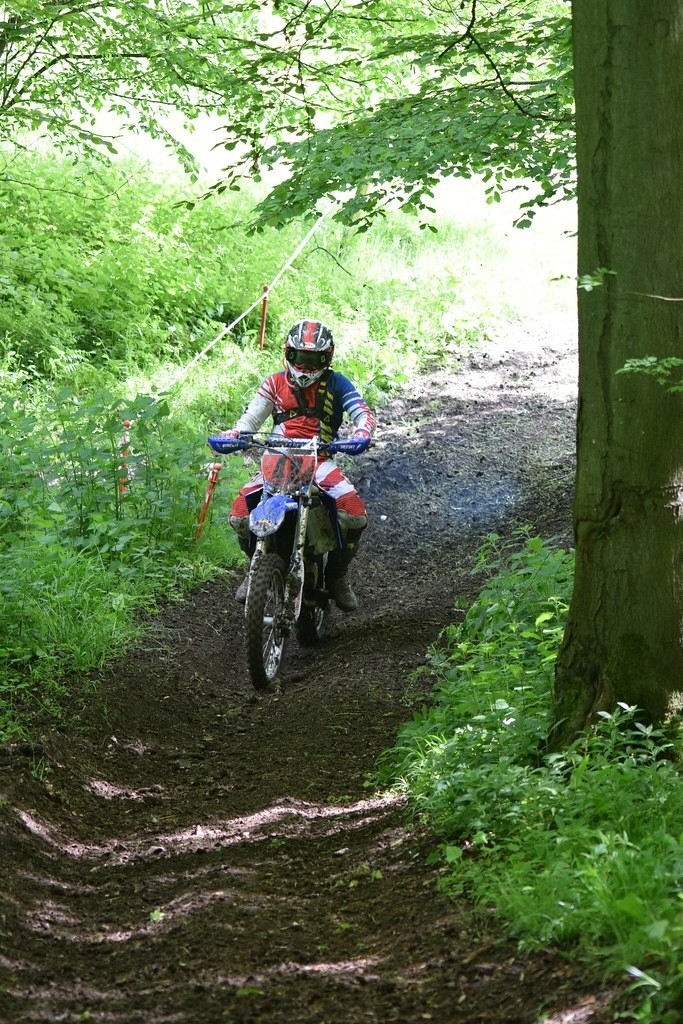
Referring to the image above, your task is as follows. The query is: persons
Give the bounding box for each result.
[231,320,376,612]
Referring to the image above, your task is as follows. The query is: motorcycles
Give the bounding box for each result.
[207,433,377,693]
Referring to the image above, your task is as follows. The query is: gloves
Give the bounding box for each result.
[219,428,240,440]
[352,431,370,440]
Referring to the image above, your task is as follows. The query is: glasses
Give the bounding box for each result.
[286,349,331,371]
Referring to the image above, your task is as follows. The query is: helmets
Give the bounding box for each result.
[284,321,335,389]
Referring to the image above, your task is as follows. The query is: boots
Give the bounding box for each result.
[325,539,359,611]
[235,536,259,604]
